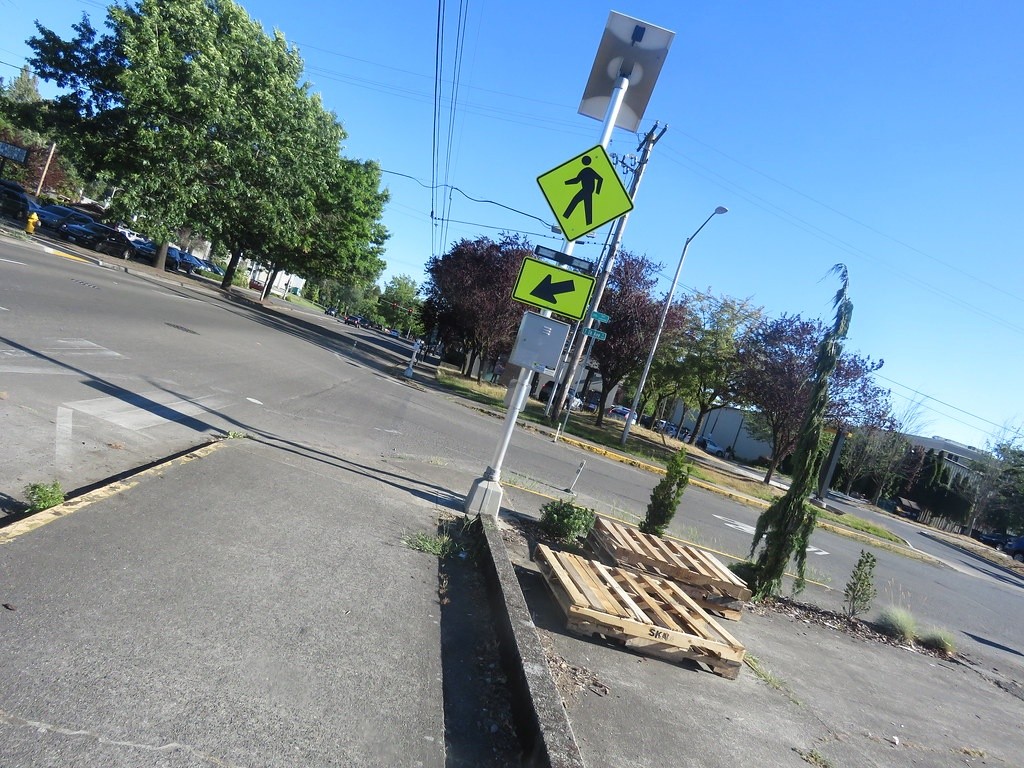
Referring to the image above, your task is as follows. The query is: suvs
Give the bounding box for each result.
[538,380,583,413]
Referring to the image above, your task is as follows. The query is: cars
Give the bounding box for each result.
[324,307,401,340]
[0,175,227,281]
[977,531,1010,551]
[1004,535,1024,564]
[607,404,724,459]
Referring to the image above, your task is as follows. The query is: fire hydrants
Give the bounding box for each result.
[23,211,42,235]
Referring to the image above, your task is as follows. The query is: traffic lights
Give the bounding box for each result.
[392,301,397,310]
[408,308,413,315]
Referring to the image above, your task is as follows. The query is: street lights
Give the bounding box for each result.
[617,205,730,445]
